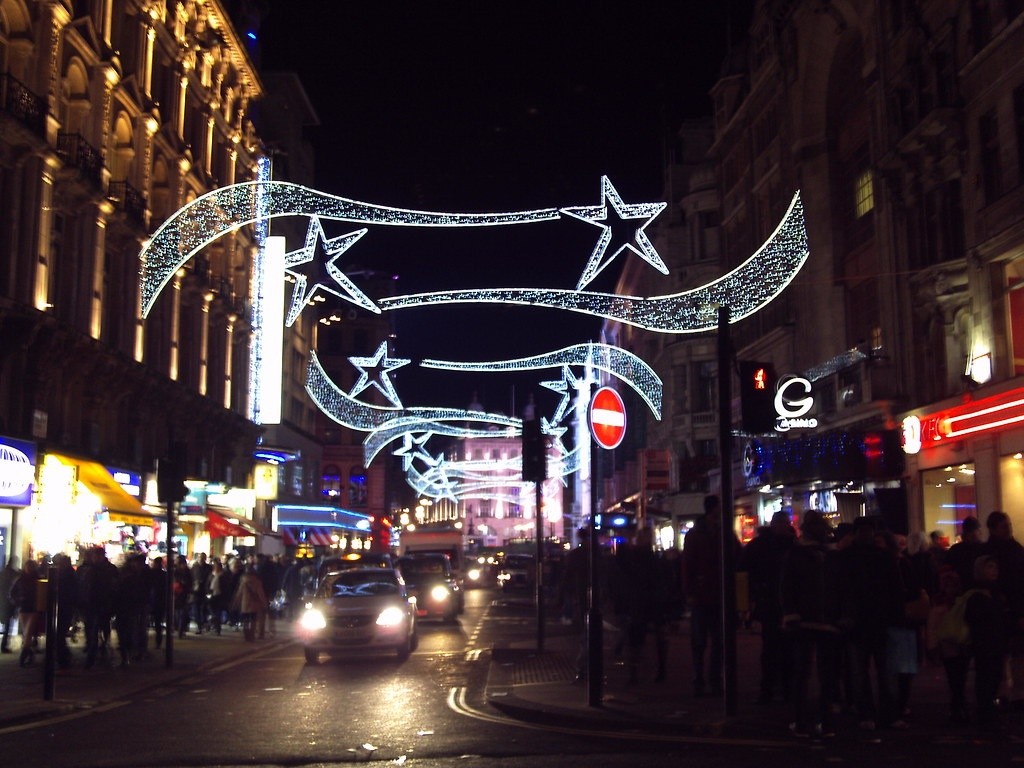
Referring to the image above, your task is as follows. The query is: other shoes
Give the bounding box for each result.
[858,732,882,743]
[789,722,808,737]
[858,720,874,730]
[891,718,911,730]
[817,723,836,738]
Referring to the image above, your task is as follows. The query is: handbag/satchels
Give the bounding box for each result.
[904,588,931,623]
[6,575,26,606]
[937,587,976,644]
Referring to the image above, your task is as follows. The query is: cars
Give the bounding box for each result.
[497,556,538,591]
[396,554,465,621]
[306,552,395,603]
[301,566,418,661]
[466,555,498,587]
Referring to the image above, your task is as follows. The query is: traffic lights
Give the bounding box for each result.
[739,359,781,435]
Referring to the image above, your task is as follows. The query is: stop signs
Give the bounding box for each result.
[587,386,630,450]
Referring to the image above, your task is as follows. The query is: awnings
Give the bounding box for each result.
[206,508,282,540]
[48,452,154,527]
[309,528,337,546]
[286,528,303,544]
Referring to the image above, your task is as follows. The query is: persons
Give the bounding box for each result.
[0,547,399,670]
[559,498,1024,732]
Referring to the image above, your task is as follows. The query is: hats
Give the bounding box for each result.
[93,546,106,554]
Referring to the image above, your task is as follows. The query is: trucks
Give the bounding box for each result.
[400,528,465,589]
